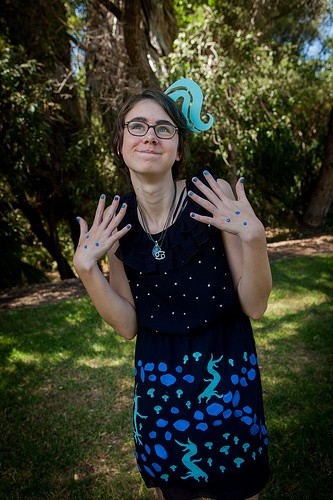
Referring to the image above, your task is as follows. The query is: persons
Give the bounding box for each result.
[72,88,273,500]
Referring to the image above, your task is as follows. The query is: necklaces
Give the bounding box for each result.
[134,179,179,261]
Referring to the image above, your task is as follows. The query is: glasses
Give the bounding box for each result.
[122,120,180,139]
